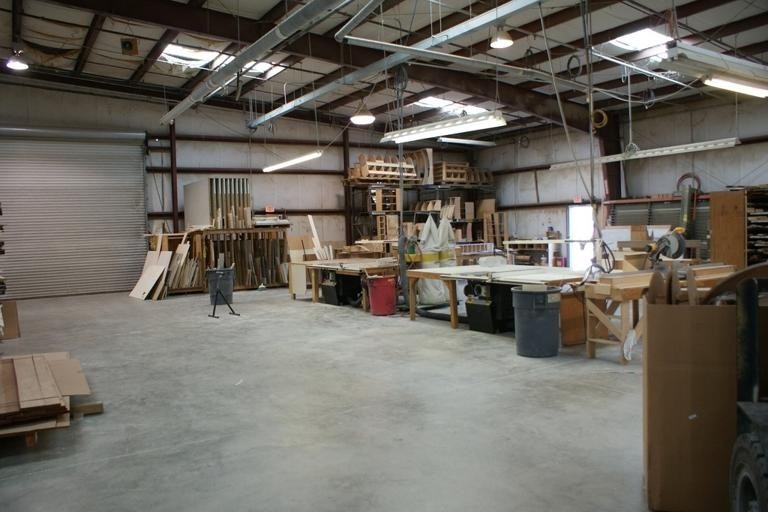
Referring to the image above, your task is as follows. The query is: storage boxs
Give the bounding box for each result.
[636,288,738,512]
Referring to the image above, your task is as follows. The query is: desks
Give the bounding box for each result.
[292,257,399,311]
[406,264,610,345]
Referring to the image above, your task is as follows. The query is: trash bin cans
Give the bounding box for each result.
[511,285,561,357]
[206,268,240,318]
[366,275,397,315]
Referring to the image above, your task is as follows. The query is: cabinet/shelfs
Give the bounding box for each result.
[341,149,562,265]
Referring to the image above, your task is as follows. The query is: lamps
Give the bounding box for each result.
[437,109,498,149]
[262,101,322,176]
[346,93,376,125]
[489,19,515,50]
[661,2,768,103]
[379,61,509,144]
[6,41,29,70]
[550,96,743,172]
